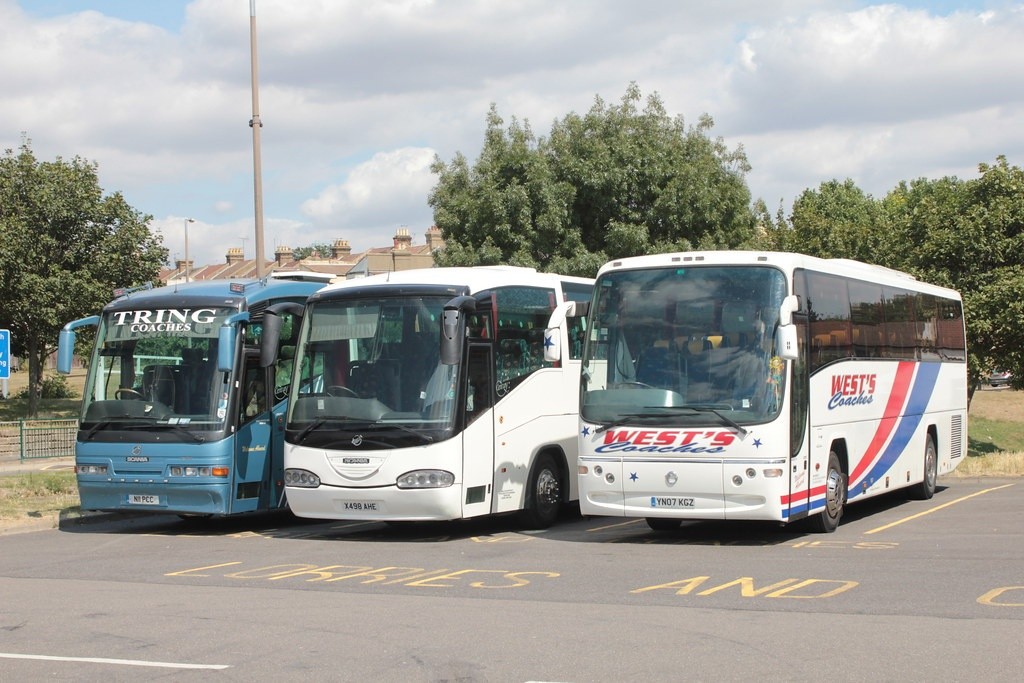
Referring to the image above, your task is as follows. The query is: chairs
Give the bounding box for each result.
[181,347,205,372]
[493,325,581,387]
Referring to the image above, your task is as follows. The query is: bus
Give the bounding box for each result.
[257,264,631,527]
[543,251,970,535]
[56,277,342,528]
